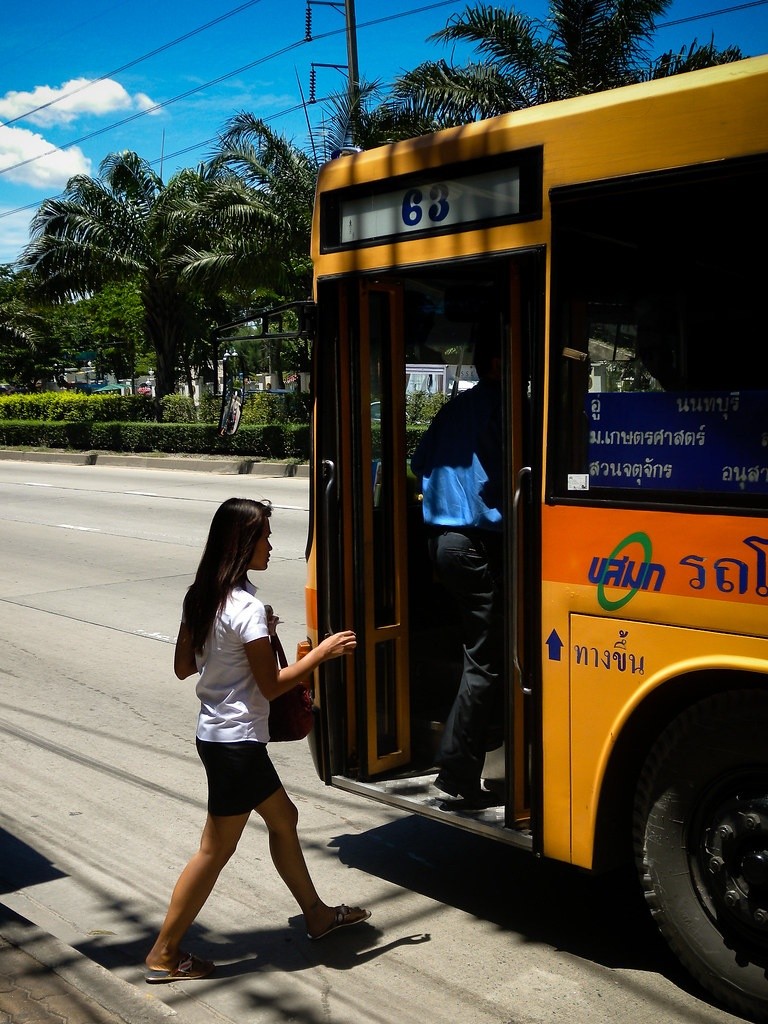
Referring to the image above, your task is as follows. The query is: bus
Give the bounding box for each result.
[211,46,768,1024]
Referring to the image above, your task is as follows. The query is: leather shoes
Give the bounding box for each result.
[434,771,495,804]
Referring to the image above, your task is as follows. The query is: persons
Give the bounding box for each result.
[145,497,371,981]
[411,331,532,804]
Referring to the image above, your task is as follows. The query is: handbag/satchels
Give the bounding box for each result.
[268,683,315,742]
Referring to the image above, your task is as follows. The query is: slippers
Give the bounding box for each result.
[306,905,372,940]
[143,954,216,983]
